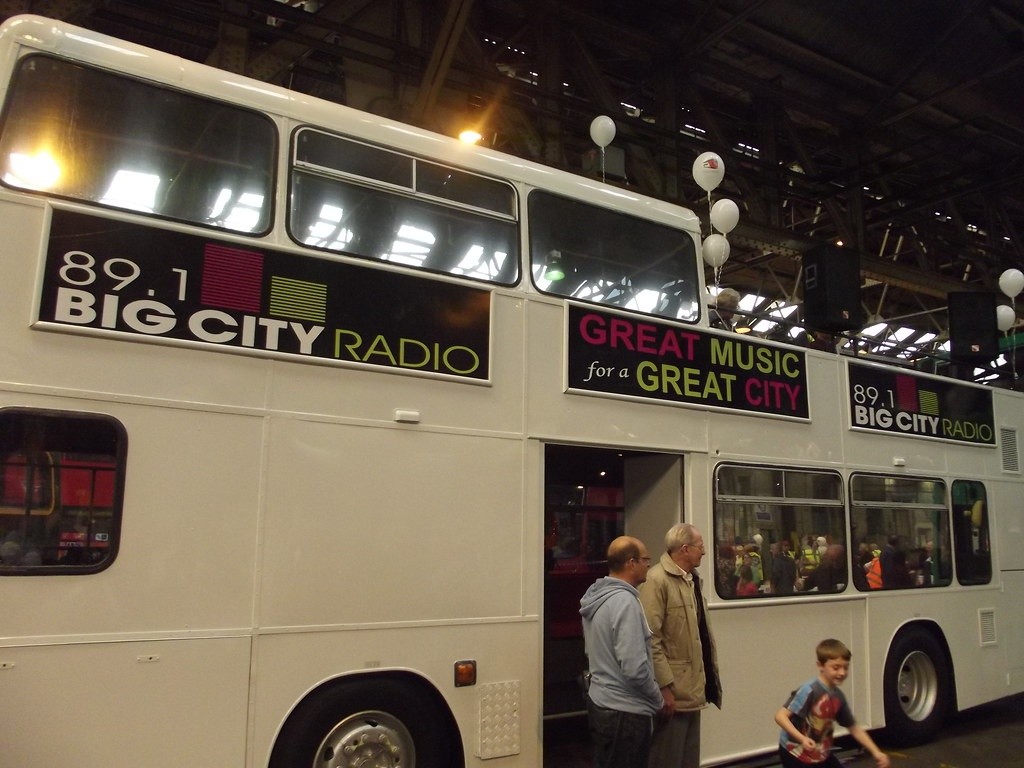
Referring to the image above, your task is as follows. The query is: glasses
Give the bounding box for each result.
[628,553,652,564]
[681,543,705,553]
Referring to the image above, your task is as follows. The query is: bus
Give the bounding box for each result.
[0,12,1024,768]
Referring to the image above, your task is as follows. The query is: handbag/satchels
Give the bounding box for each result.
[576,670,591,702]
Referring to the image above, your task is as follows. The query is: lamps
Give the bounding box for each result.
[735,321,751,334]
[543,250,565,281]
[858,346,867,355]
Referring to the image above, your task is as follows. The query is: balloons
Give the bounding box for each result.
[691,151,725,194]
[709,198,740,235]
[589,114,617,148]
[995,268,1024,332]
[702,232,731,271]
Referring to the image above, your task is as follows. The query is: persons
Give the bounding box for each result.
[0,529,42,566]
[715,532,936,599]
[773,638,890,768]
[578,533,666,768]
[795,329,841,349]
[632,522,723,768]
[709,288,739,332]
